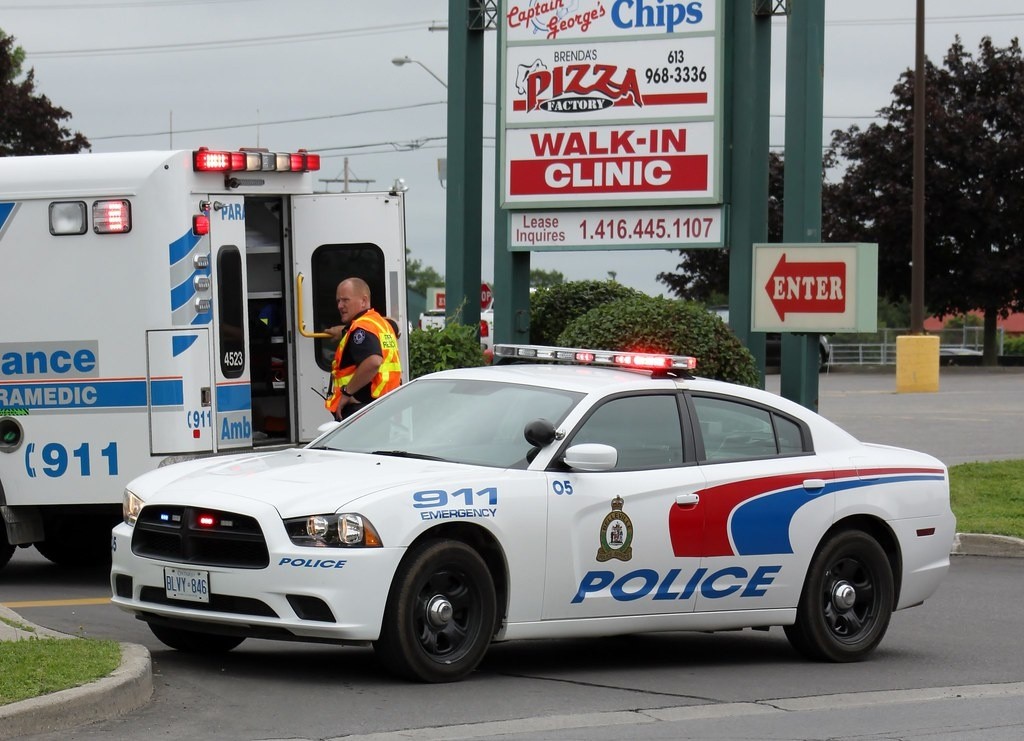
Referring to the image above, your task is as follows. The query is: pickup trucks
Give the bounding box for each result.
[418,286,551,362]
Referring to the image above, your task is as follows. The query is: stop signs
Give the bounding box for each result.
[480,282,494,311]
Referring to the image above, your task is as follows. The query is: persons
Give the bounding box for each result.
[323,278,402,420]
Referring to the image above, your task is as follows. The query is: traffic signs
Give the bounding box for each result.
[750,240,876,334]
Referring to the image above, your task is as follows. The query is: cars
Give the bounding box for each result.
[939,347,983,355]
[765,333,831,372]
[104,343,962,666]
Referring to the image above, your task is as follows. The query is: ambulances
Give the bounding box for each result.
[1,142,414,581]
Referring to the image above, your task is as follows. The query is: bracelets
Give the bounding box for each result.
[341,385,352,397]
